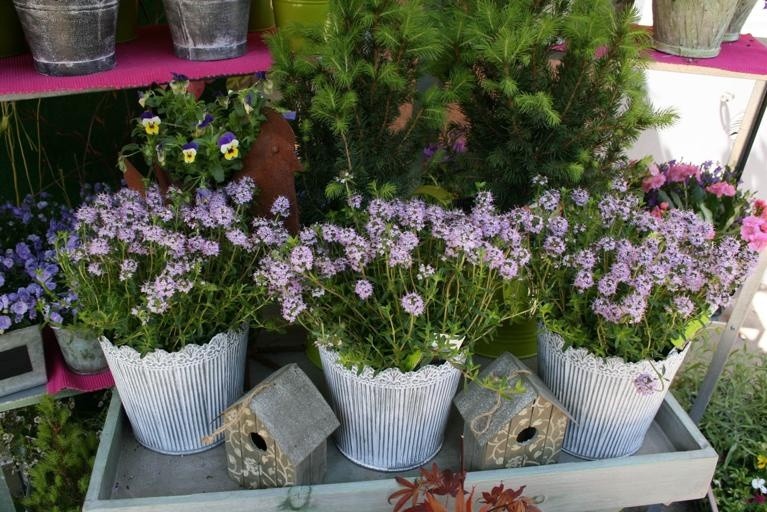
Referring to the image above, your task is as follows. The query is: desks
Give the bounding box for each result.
[83,389,723,512]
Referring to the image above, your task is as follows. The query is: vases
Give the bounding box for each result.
[162,0,335,62]
[96,322,250,456]
[0,321,108,397]
[650,1,758,59]
[0,0,142,77]
[316,342,470,473]
[536,319,693,461]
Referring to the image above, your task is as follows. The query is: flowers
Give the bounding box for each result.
[0,88,294,359]
[502,161,766,365]
[260,185,526,374]
